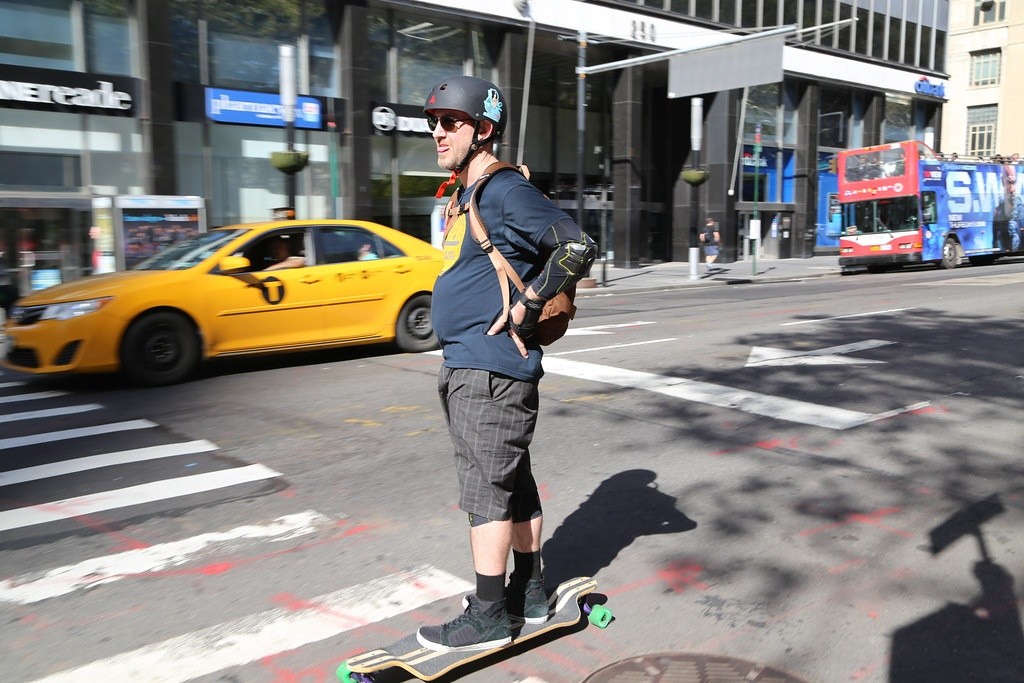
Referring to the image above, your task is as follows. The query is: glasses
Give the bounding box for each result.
[427,113,471,132]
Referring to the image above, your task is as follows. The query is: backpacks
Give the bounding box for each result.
[441,161,577,346]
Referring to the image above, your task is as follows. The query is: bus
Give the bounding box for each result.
[837,139,1023,270]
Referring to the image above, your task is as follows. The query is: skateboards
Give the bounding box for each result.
[336,575,613,683]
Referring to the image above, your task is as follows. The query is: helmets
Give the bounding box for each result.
[423,75,507,143]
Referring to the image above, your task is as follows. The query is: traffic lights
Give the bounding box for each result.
[828,158,837,175]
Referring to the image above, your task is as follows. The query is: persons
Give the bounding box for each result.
[952,152,1020,164]
[261,238,304,270]
[416,76,598,653]
[699,218,720,269]
[993,165,1024,253]
[358,244,378,259]
[860,152,905,171]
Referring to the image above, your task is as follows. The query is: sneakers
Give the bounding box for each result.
[462,572,550,625]
[416,593,511,651]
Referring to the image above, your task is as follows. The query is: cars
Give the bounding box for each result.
[1,219,442,388]
